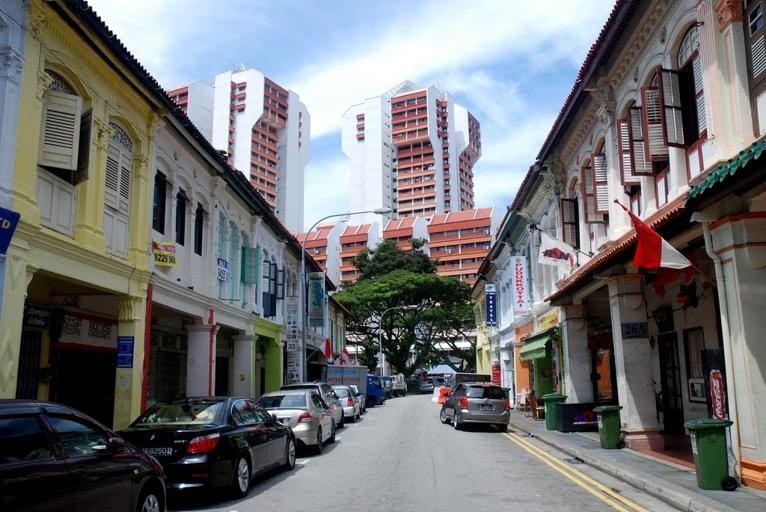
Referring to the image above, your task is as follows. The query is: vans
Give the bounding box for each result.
[280,380,345,435]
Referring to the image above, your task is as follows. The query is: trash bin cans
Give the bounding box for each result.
[541,394,568,430]
[593,406,625,449]
[502,388,510,399]
[683,418,738,491]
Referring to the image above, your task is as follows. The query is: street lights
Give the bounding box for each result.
[378,304,419,376]
[300,206,393,382]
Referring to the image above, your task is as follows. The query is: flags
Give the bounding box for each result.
[341,347,352,363]
[538,232,578,268]
[627,210,694,273]
[318,337,332,364]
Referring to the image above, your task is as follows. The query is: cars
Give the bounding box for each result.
[417,381,435,394]
[351,384,367,412]
[255,389,337,454]
[0,394,171,512]
[332,384,361,423]
[439,380,512,432]
[114,394,298,499]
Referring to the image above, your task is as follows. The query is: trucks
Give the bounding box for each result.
[308,361,408,408]
